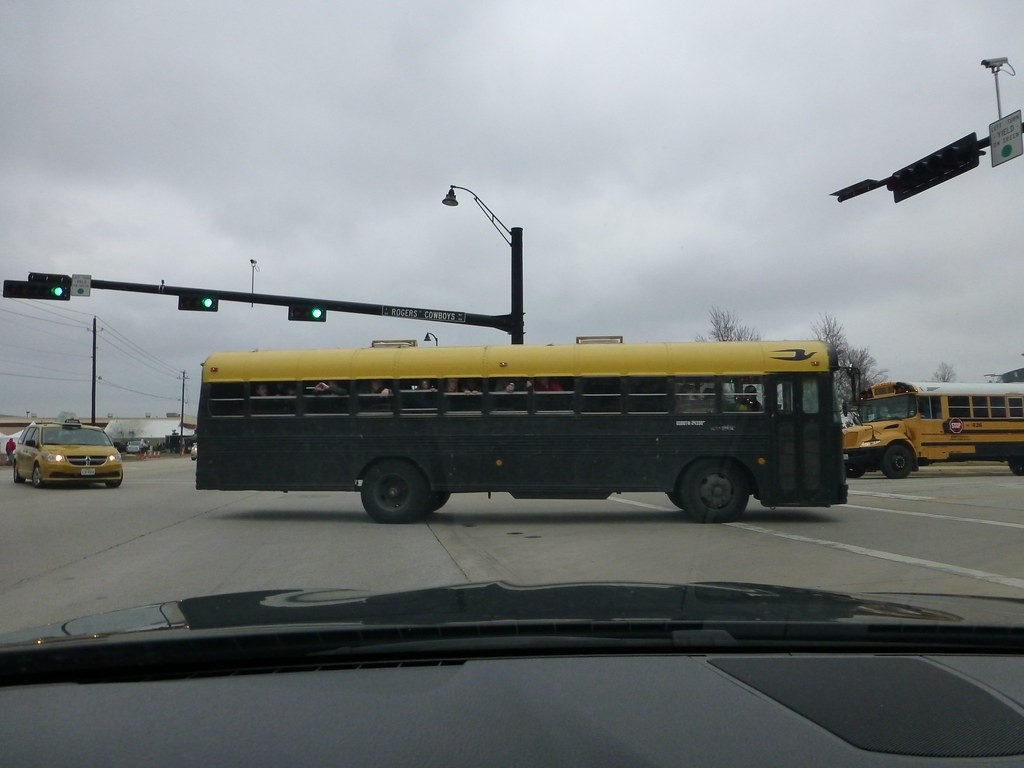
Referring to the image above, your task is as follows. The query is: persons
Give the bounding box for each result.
[419,379,480,394]
[744,384,761,411]
[678,383,714,399]
[255,384,269,396]
[141,440,148,453]
[314,380,347,395]
[494,377,563,392]
[366,380,392,397]
[6,438,16,465]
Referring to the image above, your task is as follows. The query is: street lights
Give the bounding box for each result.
[444,183,524,344]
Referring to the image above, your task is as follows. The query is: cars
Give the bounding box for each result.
[126,441,140,454]
[12,417,124,488]
[190,443,198,460]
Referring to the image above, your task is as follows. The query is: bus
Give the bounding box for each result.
[842,381,1024,479]
[195,342,853,522]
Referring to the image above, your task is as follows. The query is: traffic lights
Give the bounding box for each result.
[178,294,219,313]
[2,280,71,302]
[886,130,980,204]
[288,305,328,323]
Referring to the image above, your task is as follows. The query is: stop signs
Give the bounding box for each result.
[949,417,965,434]
